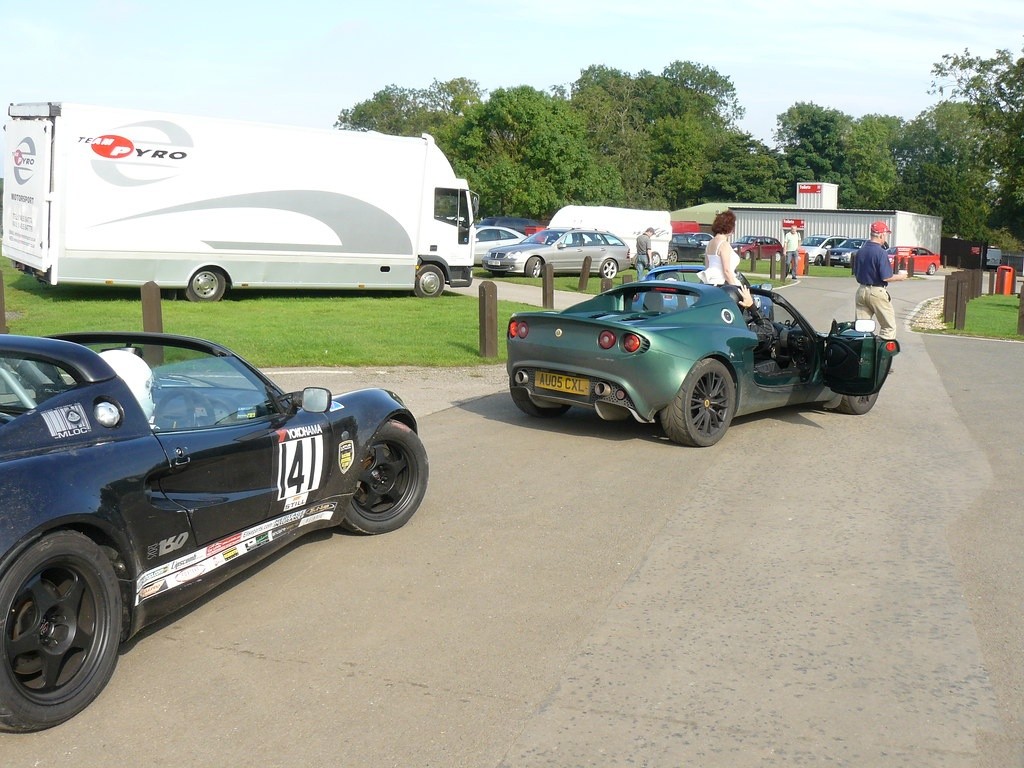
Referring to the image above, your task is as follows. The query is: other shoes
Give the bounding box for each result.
[792,276,797,279]
[786,274,787,276]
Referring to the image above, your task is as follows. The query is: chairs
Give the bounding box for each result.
[643,291,664,311]
[579,238,601,246]
[546,236,557,244]
[754,359,781,373]
[36,384,75,404]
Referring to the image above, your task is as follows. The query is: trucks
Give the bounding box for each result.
[545,205,673,270]
[1,98,484,304]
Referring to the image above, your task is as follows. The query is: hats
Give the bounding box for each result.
[646,228,656,235]
[871,221,892,234]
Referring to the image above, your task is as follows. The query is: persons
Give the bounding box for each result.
[696,210,742,285]
[98,351,159,430]
[784,225,801,279]
[719,285,789,363]
[854,221,896,373]
[637,227,654,281]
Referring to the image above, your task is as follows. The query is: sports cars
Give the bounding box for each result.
[632,265,775,319]
[506,279,902,448]
[0,331,430,734]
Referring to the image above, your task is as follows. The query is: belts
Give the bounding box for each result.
[639,254,648,255]
[861,282,884,287]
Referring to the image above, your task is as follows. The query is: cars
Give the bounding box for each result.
[729,235,783,263]
[475,216,546,267]
[481,226,632,279]
[684,231,715,246]
[884,244,941,275]
[668,234,707,263]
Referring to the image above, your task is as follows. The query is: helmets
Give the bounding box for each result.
[721,286,745,314]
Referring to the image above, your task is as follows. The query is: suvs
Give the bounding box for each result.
[830,237,892,269]
[800,234,855,265]
